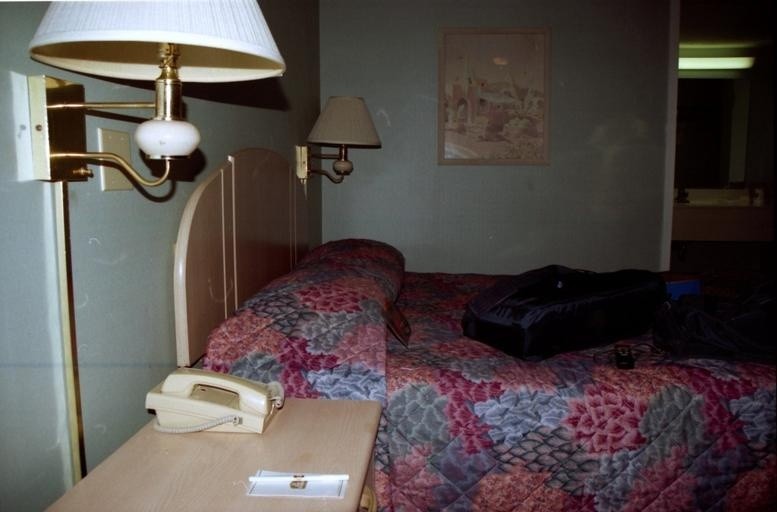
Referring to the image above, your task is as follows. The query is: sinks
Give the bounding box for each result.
[692,198,758,207]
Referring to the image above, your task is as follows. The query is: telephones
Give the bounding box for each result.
[144,368,275,434]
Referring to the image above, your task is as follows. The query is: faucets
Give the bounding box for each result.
[675,184,689,204]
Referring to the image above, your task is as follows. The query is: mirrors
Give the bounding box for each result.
[674,77,751,190]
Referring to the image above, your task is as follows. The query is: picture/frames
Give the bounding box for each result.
[435,24,552,171]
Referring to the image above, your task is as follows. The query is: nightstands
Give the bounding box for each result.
[34,392,383,511]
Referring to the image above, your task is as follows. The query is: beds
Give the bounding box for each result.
[168,139,777,511]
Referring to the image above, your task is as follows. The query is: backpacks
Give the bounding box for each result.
[459,263,671,362]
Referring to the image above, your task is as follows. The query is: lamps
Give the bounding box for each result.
[26,1,287,188]
[301,94,382,184]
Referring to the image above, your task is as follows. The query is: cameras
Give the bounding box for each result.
[615,343,633,369]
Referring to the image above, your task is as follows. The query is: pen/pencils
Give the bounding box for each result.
[248,474,351,482]
